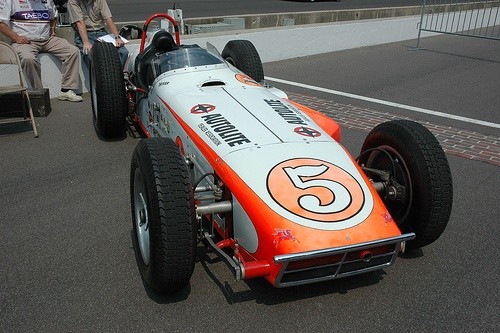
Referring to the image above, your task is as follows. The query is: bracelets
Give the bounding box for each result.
[115,35,120,39]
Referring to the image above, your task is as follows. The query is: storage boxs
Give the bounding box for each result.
[26,88,52,117]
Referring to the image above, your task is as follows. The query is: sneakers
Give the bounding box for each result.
[58,90,83,101]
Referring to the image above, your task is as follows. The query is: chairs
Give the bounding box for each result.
[134,31,183,90]
[0,41,39,138]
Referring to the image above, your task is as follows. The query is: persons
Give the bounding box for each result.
[67,0,129,72]
[0,0,83,102]
